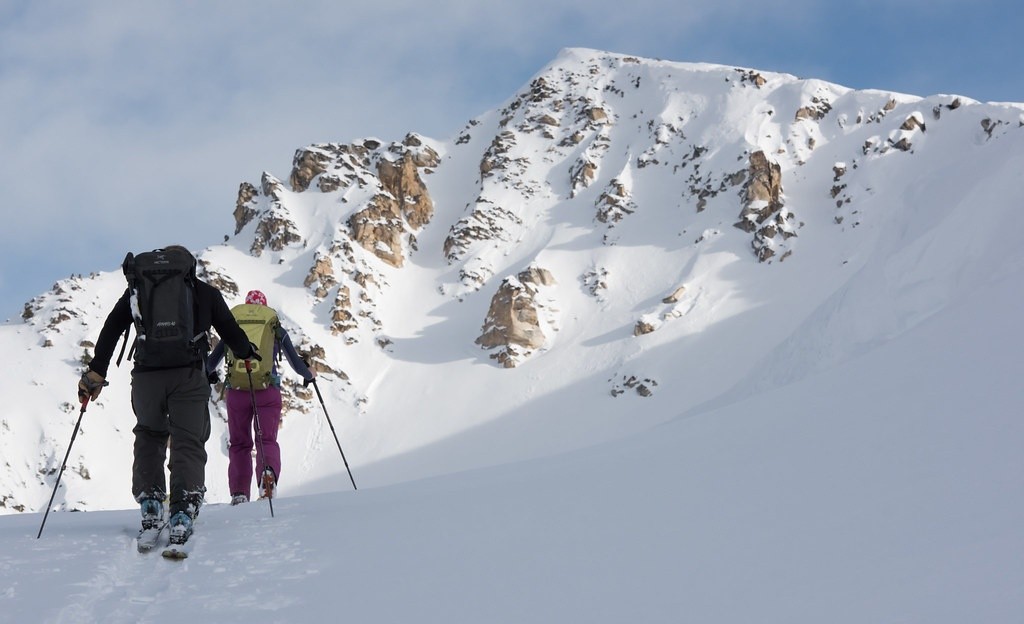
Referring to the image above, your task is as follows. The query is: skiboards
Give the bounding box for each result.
[231,467,276,506]
[135,493,189,559]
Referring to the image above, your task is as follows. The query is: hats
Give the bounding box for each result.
[246,290,267,306]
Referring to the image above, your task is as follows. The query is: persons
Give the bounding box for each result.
[207,290,318,505]
[77,276,263,558]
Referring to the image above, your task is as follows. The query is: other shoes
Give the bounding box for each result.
[259,466,277,499]
[169,512,193,545]
[141,498,164,530]
[230,493,248,506]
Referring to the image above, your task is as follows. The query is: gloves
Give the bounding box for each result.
[238,342,260,363]
[77,369,108,403]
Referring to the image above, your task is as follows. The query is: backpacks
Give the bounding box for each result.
[122,245,208,371]
[217,304,281,392]
